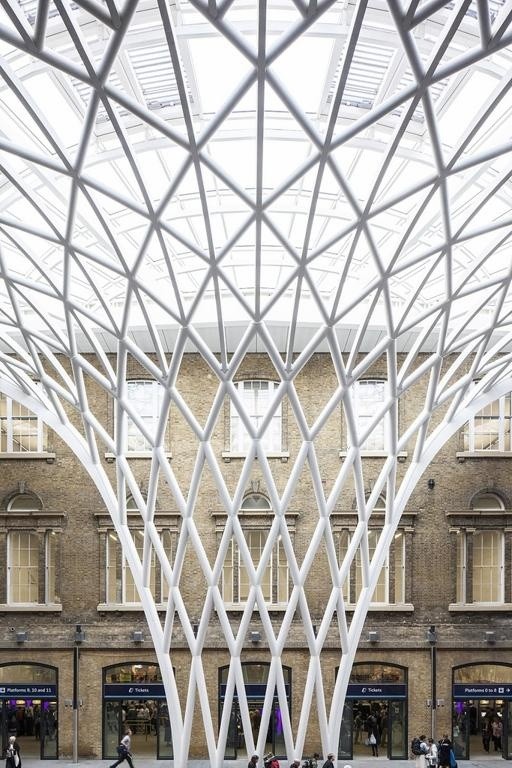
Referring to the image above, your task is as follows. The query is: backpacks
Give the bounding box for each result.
[410,737,425,755]
[369,733,376,745]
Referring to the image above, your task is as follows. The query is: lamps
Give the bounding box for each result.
[134,631,144,643]
[16,633,26,643]
[251,631,260,643]
[368,632,378,643]
[485,632,496,643]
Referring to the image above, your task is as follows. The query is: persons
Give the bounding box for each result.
[415,735,429,768]
[108,699,172,747]
[470,702,504,754]
[248,752,352,768]
[425,738,438,768]
[7,704,57,742]
[355,705,393,758]
[4,736,22,768]
[438,733,452,768]
[110,728,136,768]
